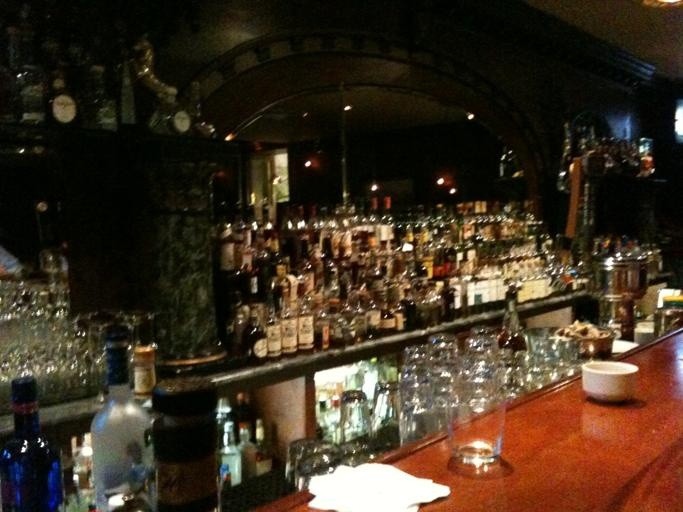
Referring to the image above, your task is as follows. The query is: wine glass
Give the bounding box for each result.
[0,249,108,415]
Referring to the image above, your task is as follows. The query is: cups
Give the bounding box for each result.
[285,326,578,491]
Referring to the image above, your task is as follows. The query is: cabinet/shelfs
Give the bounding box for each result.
[0,291,602,512]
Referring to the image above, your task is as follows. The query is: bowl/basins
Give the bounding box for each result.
[581,361,639,404]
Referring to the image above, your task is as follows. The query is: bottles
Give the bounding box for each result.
[0,324,180,512]
[214,392,273,488]
[562,126,653,177]
[590,235,683,340]
[216,197,585,367]
